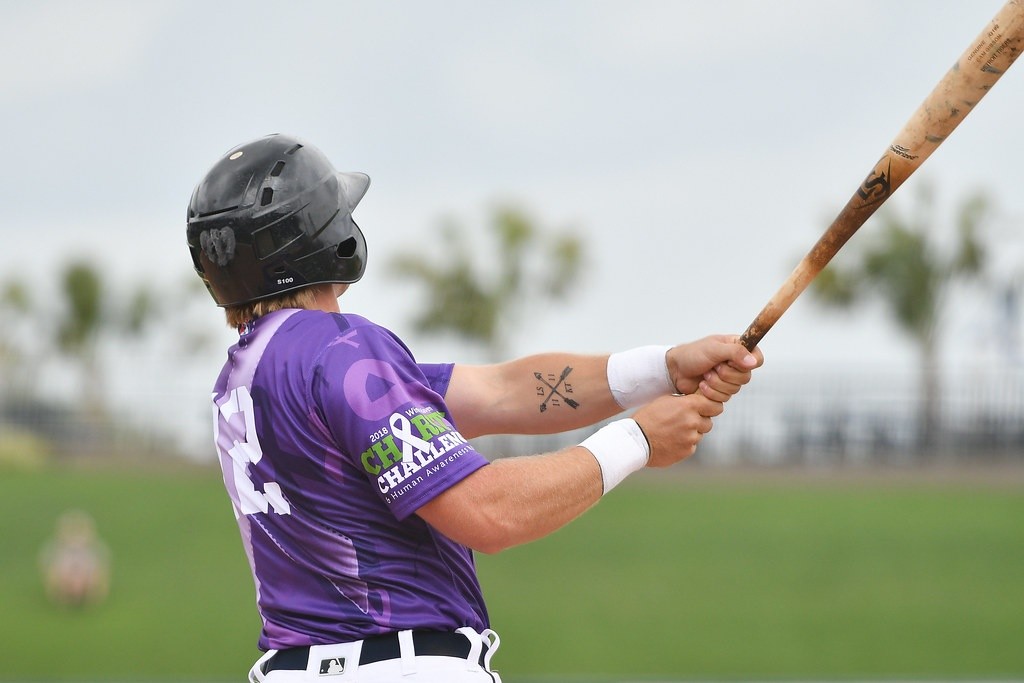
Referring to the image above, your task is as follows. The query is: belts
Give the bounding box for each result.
[260,631,489,676]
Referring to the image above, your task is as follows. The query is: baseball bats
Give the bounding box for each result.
[692,0,1024,401]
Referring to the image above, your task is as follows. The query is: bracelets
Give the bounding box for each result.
[606,344,677,410]
[576,417,650,498]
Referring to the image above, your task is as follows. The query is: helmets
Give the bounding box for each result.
[186,134,371,307]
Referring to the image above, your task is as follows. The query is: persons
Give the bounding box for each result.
[185,132,764,683]
